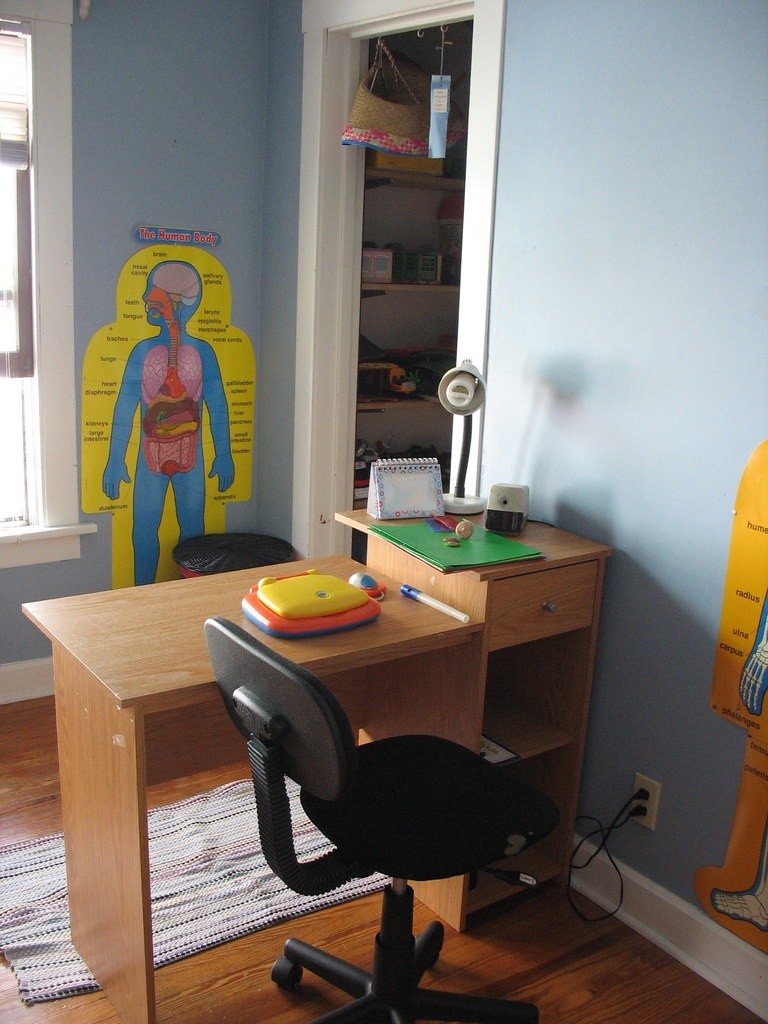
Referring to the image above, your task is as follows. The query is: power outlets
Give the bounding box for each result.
[627,772,662,833]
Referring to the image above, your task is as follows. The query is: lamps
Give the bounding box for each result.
[436,358,488,516]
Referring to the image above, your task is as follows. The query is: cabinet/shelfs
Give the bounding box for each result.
[21,509,613,1024]
[353,168,466,509]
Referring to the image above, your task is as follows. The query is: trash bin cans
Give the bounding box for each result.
[172,530,299,580]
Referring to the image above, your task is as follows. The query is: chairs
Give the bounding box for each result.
[204,617,561,1024]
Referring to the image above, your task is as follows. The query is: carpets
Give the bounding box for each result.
[0,778,392,1007]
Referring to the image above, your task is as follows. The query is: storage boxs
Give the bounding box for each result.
[361,248,393,284]
